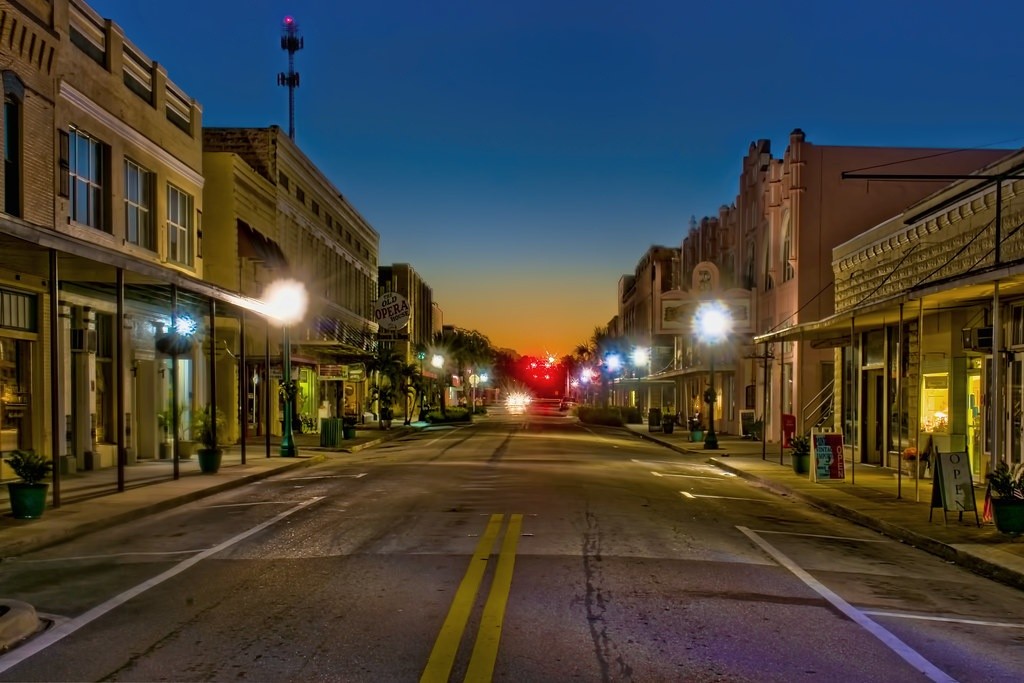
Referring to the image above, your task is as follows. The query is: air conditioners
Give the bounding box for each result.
[962,326,993,351]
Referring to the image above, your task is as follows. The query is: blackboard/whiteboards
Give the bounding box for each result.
[739,410,756,436]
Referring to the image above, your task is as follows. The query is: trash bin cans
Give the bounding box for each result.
[319,418,341,447]
[649,408,661,426]
[662,414,675,434]
[342,418,357,438]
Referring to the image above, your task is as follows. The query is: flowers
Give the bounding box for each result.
[903,446,922,460]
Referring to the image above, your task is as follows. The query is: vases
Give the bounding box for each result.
[906,460,927,479]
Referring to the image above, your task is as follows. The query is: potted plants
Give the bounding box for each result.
[788,435,810,475]
[157,401,227,475]
[984,460,1024,535]
[662,413,677,434]
[689,420,706,442]
[342,417,357,440]
[380,407,394,428]
[3,447,53,519]
